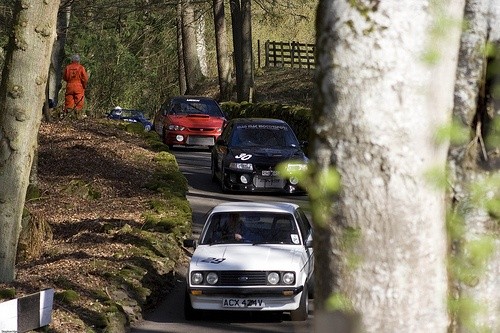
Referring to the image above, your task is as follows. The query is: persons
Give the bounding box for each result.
[64,55,88,113]
[261,129,276,145]
[219,214,246,243]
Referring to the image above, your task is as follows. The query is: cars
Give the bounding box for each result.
[150,95,228,148]
[211,118,310,197]
[107,108,152,132]
[182,202,316,321]
